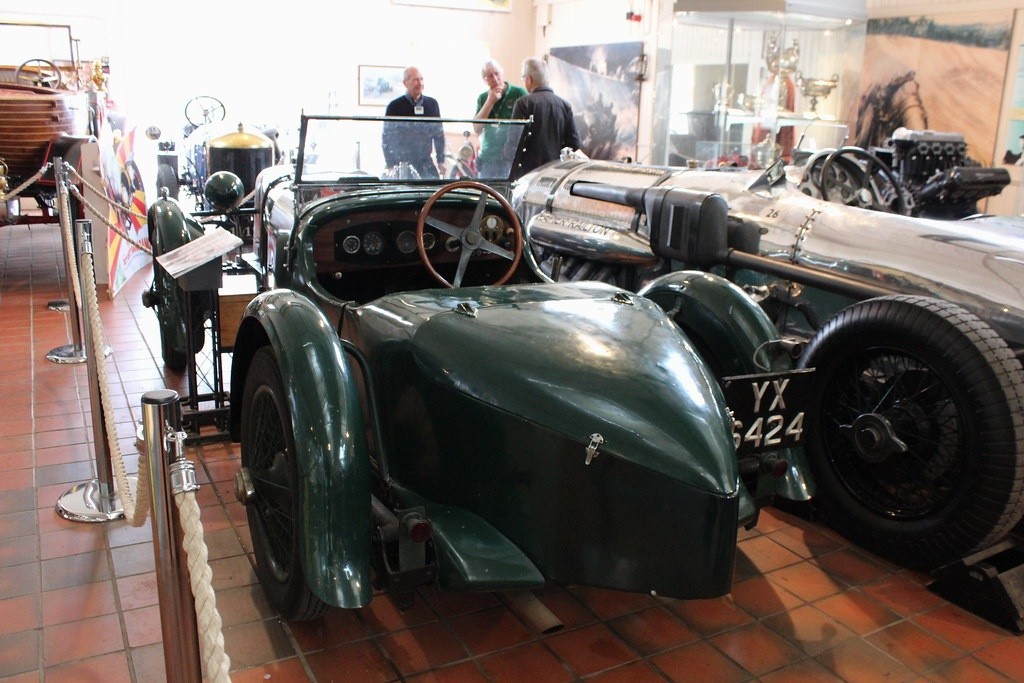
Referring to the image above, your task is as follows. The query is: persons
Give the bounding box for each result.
[381,67,447,180]
[472,57,529,181]
[502,57,580,182]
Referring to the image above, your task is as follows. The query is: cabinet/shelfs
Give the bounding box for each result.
[668,0,868,175]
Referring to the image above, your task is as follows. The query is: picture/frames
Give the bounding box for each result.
[359,65,407,107]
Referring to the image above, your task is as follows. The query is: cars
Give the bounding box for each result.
[0,16,1024,627]
